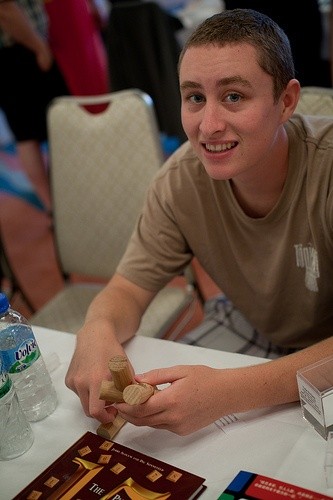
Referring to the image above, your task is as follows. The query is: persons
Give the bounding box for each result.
[45,0,110,114]
[65,9,333,436]
[1,0,60,216]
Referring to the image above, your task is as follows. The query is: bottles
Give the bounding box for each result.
[0,360,34,460]
[0,292,57,422]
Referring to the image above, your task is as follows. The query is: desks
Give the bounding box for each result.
[1,321,333,499]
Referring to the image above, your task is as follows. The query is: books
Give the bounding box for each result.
[11,430,207,500]
[217,471,333,500]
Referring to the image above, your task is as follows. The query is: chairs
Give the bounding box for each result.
[29,89,206,337]
